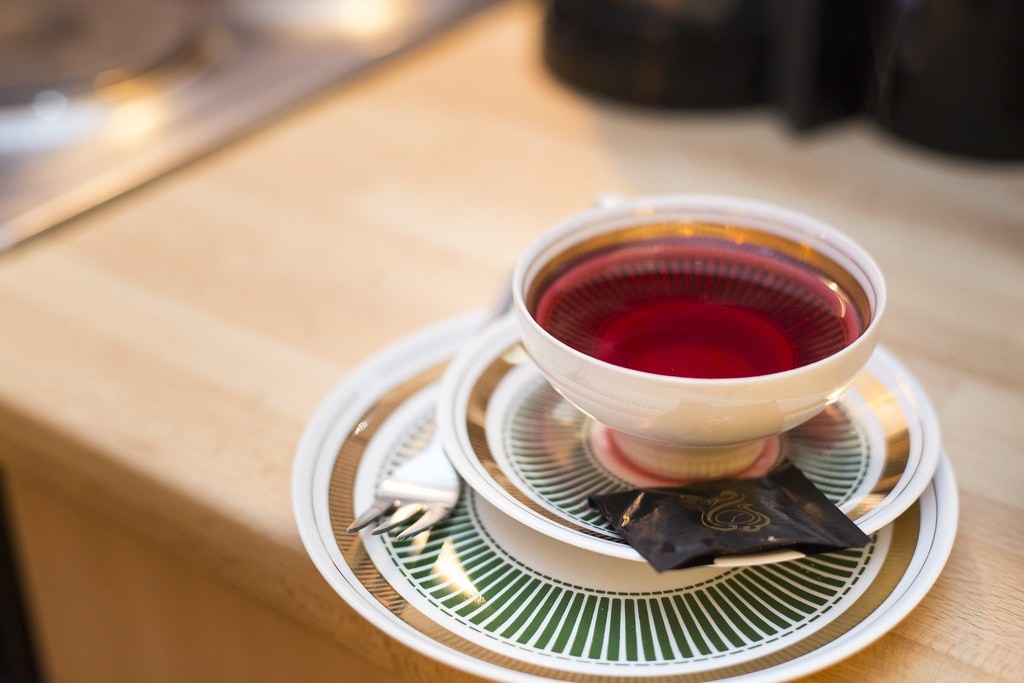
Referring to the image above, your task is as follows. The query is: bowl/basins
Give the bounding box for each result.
[519,194,887,478]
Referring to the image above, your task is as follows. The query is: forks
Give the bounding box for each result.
[347,438,461,540]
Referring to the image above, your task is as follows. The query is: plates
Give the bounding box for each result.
[438,320,939,567]
[291,311,958,683]
